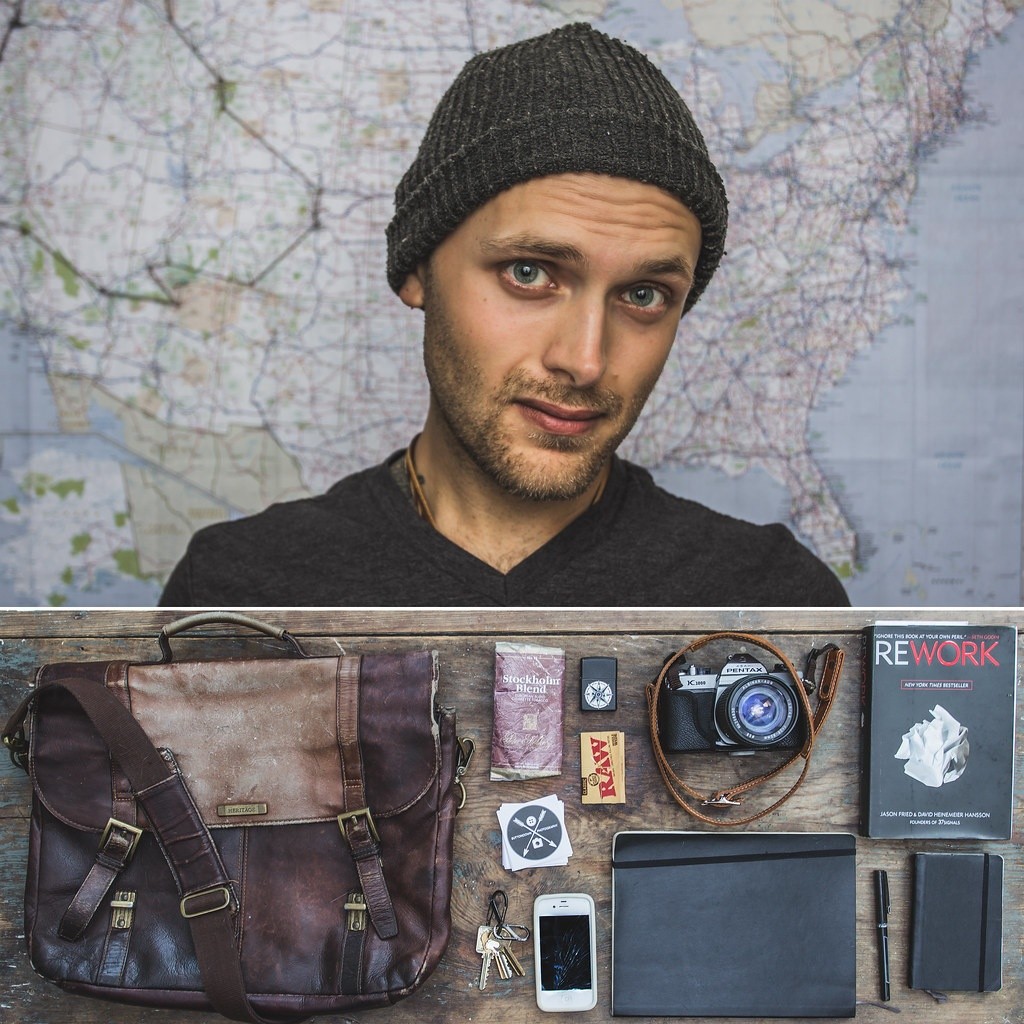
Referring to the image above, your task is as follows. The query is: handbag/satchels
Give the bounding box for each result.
[2,612,474,1023]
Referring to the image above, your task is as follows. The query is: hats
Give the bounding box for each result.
[385,24,731,325]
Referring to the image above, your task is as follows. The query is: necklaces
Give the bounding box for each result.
[406,431,603,530]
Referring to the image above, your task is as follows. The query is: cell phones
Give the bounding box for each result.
[534,893,598,1012]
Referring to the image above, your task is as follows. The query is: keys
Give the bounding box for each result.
[476,925,525,991]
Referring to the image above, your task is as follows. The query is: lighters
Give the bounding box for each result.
[582,657,618,711]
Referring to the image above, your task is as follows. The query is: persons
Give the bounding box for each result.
[156,25,849,608]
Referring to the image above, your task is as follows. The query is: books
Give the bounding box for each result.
[858,625,1016,839]
[907,852,1004,990]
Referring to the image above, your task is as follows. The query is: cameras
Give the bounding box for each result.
[658,653,807,754]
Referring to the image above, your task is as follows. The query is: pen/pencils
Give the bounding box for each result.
[872,869,891,1002]
